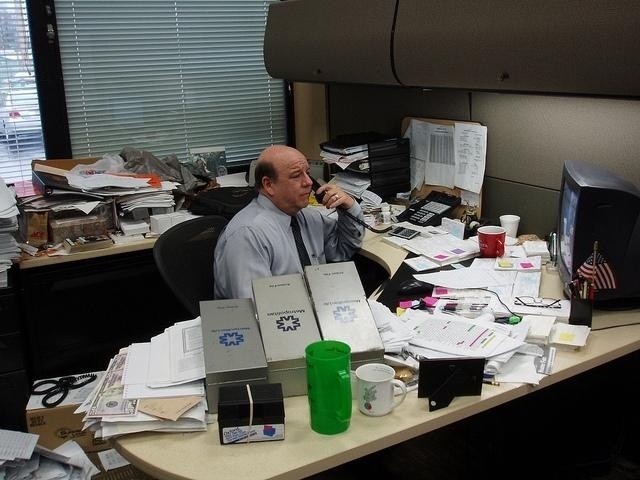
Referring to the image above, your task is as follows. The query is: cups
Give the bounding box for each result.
[355,363,406,415]
[380,212,391,225]
[478,226,506,258]
[500,215,521,242]
[568,295,593,327]
[304,340,352,436]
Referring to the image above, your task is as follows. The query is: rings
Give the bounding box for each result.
[334,195,339,200]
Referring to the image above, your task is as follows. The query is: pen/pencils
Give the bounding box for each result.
[483,380,500,386]
[569,273,595,300]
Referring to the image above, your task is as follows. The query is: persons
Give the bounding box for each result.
[197,152,226,177]
[212,144,366,314]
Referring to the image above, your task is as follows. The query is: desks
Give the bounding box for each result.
[14,184,640,479]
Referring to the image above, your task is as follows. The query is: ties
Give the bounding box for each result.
[290,217,311,270]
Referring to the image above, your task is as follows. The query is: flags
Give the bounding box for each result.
[577,250,617,290]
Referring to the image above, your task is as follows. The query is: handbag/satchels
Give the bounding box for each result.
[188,187,257,218]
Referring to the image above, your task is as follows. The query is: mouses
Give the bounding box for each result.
[398,280,433,296]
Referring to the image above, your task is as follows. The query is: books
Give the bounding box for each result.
[31,156,103,178]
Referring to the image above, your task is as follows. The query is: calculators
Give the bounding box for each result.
[388,226,421,240]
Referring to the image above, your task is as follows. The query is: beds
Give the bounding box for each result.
[153,210,226,310]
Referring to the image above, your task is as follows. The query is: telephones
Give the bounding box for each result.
[308,174,326,204]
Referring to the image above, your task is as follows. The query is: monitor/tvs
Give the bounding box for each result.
[556,160,640,309]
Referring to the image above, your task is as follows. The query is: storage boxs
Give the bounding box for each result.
[249,274,324,400]
[198,297,269,414]
[25,371,113,449]
[217,383,286,445]
[302,258,386,372]
